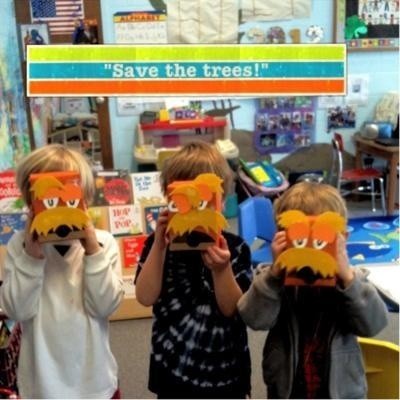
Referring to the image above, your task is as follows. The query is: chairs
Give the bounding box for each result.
[237,197,275,267]
[330,132,389,213]
[356,336,399,399]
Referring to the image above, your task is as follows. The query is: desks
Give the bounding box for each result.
[354,135,399,217]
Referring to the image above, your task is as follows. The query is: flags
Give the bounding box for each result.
[29,0,86,37]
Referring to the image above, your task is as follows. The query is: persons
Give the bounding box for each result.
[237,181,388,399]
[0,143,124,399]
[132,140,255,399]
